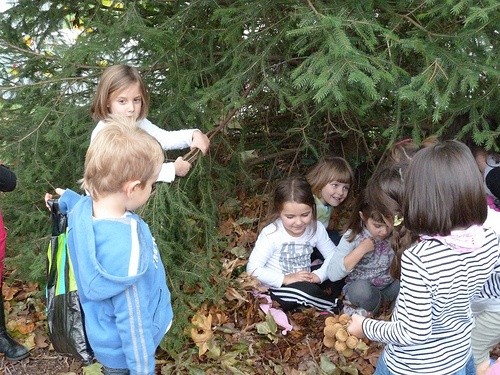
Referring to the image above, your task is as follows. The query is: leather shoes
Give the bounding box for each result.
[0,330,30,361]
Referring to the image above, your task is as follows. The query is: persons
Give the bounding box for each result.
[0,160,32,362]
[89,65,210,183]
[247,139,500,375]
[45,115,174,375]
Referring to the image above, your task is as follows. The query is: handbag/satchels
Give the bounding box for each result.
[45,198,95,364]
